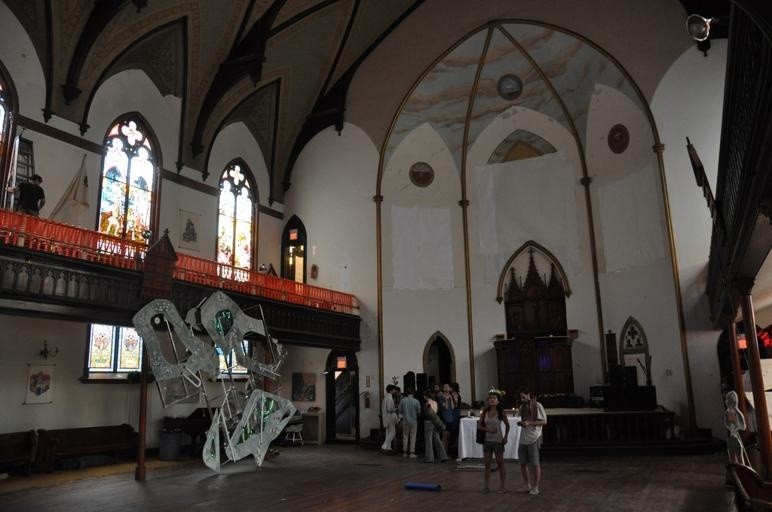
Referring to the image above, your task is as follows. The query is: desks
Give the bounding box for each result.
[458,415,523,461]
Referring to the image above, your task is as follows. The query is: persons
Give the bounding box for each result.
[515,387,549,497]
[476,391,511,494]
[5,174,48,220]
[378,380,464,465]
[722,390,747,464]
[744,395,759,449]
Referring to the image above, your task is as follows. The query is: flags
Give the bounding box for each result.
[47,154,91,226]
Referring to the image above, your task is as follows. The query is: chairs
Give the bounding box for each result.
[725,462,772,511]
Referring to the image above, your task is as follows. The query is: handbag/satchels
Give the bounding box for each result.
[475,408,486,444]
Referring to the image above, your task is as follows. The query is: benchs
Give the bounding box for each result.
[0,423,146,472]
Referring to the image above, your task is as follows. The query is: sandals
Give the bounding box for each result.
[480,487,509,494]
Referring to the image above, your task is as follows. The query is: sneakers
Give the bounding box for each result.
[514,482,541,496]
[379,444,451,464]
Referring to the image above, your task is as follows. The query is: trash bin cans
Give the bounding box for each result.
[158,429,185,461]
